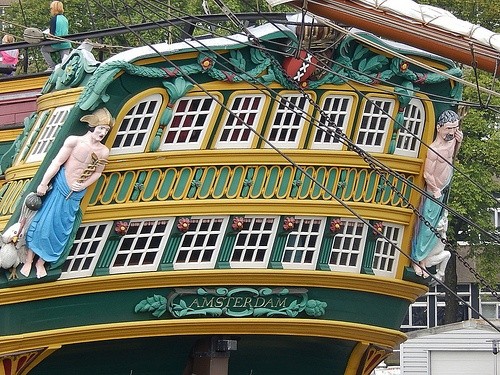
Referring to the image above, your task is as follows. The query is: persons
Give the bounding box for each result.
[0,34,20,78]
[39,0,73,73]
[424,209,452,287]
[19,107,117,280]
[412,110,464,279]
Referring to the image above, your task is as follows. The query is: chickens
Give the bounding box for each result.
[0,222,25,281]
